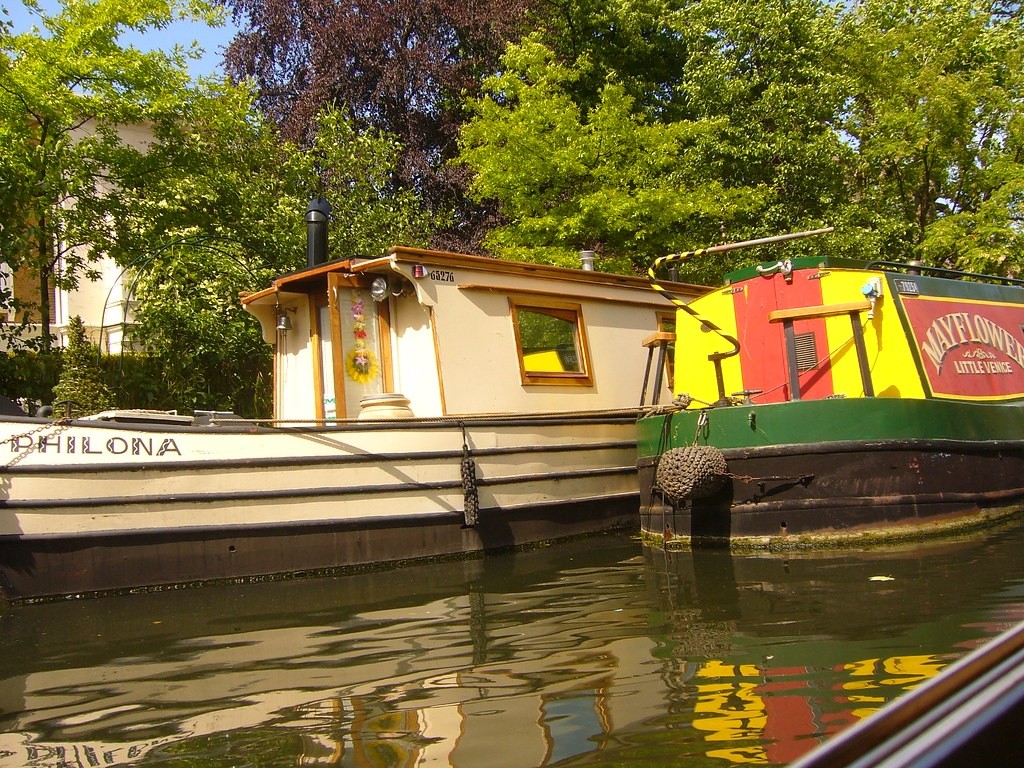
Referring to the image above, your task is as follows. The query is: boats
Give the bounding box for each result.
[639,228,1024,555]
[1,193,724,613]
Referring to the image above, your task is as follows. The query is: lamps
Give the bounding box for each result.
[372,277,415,302]
[276,308,296,331]
